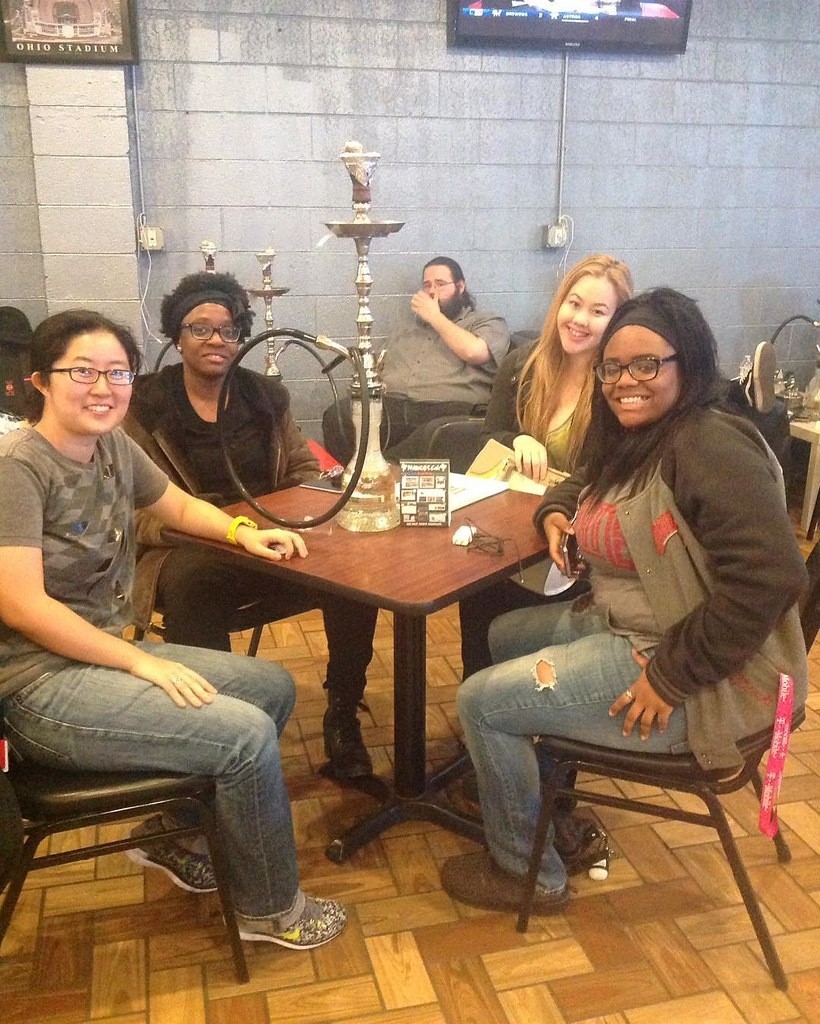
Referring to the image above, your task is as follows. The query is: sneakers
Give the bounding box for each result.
[126,815,217,893]
[556,812,598,858]
[441,851,571,915]
[223,890,347,951]
[741,342,777,413]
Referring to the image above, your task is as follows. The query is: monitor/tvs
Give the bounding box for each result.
[446,0,693,55]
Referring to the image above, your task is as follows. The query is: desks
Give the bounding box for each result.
[163,463,576,866]
[789,411,820,539]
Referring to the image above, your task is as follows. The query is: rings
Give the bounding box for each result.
[173,677,183,686]
[626,690,635,700]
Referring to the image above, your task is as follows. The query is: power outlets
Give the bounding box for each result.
[136,224,167,251]
[547,225,569,249]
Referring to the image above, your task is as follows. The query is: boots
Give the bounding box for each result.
[324,675,373,778]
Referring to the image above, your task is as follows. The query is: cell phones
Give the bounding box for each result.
[561,532,579,578]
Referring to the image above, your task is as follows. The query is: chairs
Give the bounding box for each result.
[142,584,336,656]
[1,699,252,983]
[514,567,803,988]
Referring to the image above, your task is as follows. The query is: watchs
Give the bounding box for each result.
[226,515,258,546]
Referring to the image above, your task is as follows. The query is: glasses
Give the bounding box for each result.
[593,352,677,383]
[180,321,243,342]
[46,367,134,386]
[424,281,456,289]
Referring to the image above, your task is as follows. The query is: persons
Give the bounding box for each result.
[119,271,381,778]
[442,287,809,908]
[729,341,792,514]
[322,257,510,469]
[0,309,349,950]
[458,253,635,681]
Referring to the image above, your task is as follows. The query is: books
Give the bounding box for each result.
[299,475,342,494]
[400,458,452,527]
[395,472,509,512]
